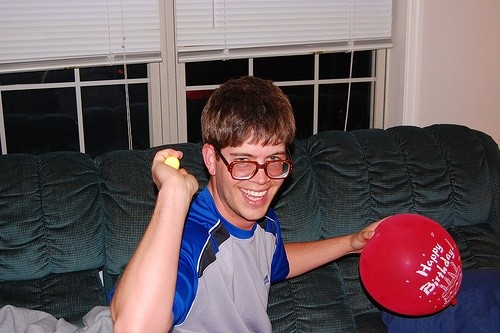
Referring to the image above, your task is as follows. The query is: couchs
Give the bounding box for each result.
[0,124,500,333]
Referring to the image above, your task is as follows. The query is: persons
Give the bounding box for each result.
[110,74,393,333]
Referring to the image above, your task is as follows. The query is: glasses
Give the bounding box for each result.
[214,148,293,180]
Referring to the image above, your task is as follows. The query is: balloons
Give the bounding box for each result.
[359,212,464,317]
